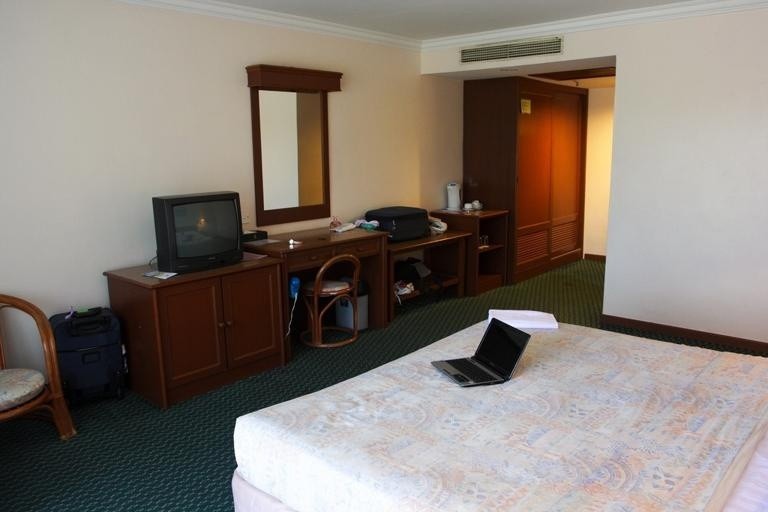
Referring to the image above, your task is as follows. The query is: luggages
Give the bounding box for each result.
[365,207,431,242]
[49,306,130,409]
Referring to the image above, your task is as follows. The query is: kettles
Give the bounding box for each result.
[447,183,461,212]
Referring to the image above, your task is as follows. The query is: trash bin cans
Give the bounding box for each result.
[335,281,368,331]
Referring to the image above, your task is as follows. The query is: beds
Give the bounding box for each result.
[231,320,768,512]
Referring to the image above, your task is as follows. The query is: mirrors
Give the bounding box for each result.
[245,64,343,227]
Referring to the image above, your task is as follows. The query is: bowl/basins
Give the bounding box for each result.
[464,200,484,210]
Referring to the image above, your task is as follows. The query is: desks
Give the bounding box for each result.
[240,226,391,364]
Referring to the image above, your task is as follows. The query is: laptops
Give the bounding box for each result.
[429,317,531,387]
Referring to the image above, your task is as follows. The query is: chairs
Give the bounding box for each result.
[299,253,361,349]
[0,294,77,441]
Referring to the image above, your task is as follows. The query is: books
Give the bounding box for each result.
[488,308,560,329]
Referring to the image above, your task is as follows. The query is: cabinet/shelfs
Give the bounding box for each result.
[103,252,286,410]
[388,209,509,322]
[463,76,588,285]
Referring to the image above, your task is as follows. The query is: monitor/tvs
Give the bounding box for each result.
[152,190,244,275]
